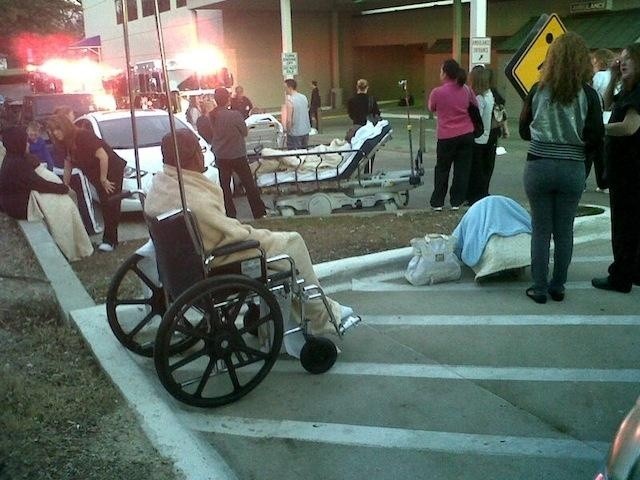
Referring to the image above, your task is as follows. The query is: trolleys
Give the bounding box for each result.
[257,116,426,215]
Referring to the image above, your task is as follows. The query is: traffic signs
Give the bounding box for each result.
[471,37,492,64]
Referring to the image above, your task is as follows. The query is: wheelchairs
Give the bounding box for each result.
[105,188,360,409]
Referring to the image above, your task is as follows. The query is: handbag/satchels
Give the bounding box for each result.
[491,103,508,129]
[406,237,461,286]
[468,103,484,138]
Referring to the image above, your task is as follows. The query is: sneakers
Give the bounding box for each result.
[430,206,459,212]
[98,243,115,252]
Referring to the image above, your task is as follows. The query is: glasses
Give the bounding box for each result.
[194,146,207,153]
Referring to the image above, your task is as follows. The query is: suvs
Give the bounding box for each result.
[20,93,103,126]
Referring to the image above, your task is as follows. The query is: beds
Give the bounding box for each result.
[247,119,393,192]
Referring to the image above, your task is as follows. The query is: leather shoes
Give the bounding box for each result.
[526,287,547,304]
[590,277,631,293]
[548,286,565,301]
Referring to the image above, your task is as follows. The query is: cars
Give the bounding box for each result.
[71,108,219,215]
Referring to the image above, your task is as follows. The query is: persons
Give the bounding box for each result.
[346,78,381,125]
[283,77,312,150]
[308,80,323,131]
[143,128,353,339]
[185,85,267,221]
[428,58,508,212]
[518,30,604,304]
[583,42,639,294]
[0,105,128,263]
